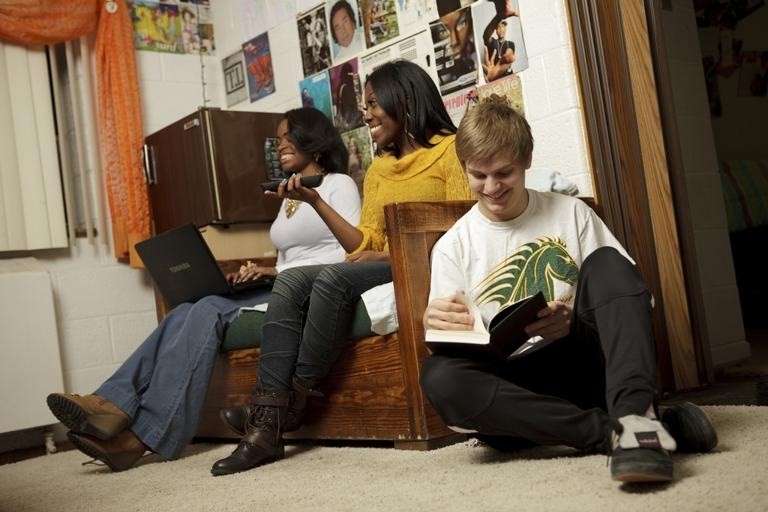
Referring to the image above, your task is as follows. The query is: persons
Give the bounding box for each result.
[436,10,472,60]
[335,63,363,130]
[370,7,388,38]
[44,108,363,473]
[479,1,522,83]
[208,59,476,479]
[305,20,328,72]
[302,87,314,110]
[417,94,717,488]
[330,0,366,67]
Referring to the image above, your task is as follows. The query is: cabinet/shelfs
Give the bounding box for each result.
[144,106,285,234]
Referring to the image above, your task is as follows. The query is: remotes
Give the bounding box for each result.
[260,174,323,191]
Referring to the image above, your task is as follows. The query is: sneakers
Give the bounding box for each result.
[610,414,676,484]
[660,401,717,456]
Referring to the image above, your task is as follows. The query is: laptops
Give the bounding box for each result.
[134,222,276,309]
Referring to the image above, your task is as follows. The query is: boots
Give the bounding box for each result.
[219,378,327,437]
[211,376,290,476]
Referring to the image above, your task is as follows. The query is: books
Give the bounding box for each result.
[424,288,550,371]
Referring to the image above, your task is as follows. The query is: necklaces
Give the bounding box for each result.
[283,167,326,219]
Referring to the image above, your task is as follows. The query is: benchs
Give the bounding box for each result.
[154,196,597,451]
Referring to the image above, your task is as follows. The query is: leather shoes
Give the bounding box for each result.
[66,430,147,472]
[47,393,132,442]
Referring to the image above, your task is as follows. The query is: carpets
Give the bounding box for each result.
[1,404,767,511]
